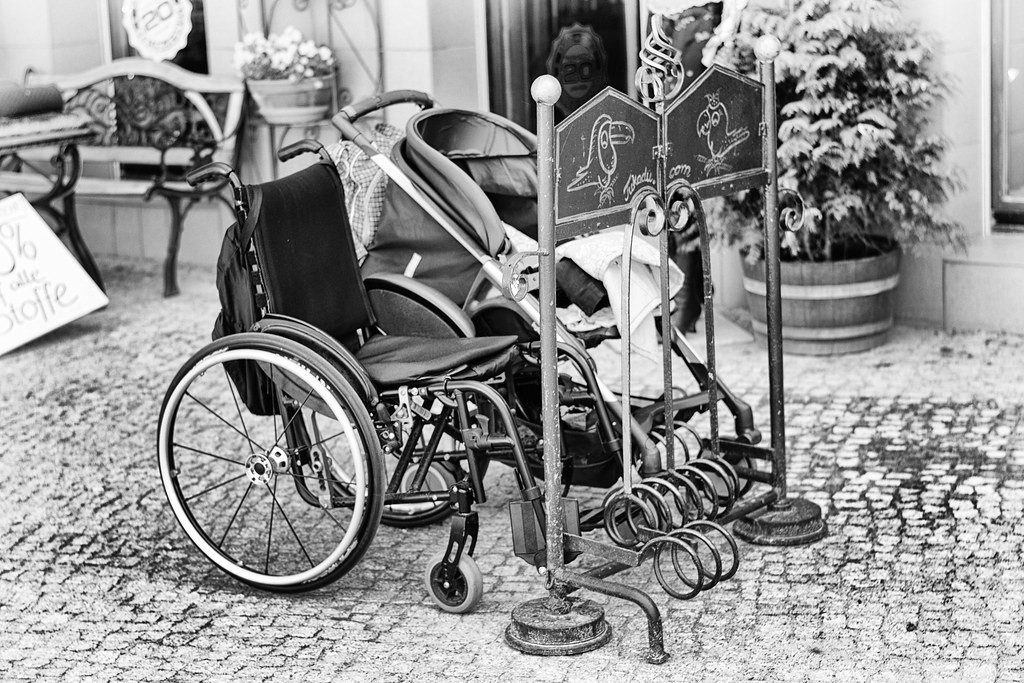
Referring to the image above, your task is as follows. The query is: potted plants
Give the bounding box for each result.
[702,0,967,355]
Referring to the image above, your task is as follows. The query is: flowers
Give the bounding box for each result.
[236,26,336,85]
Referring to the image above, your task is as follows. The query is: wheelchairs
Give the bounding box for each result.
[152,137,644,617]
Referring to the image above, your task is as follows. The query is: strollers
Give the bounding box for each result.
[332,89,696,489]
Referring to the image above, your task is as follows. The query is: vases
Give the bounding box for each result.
[246,75,333,123]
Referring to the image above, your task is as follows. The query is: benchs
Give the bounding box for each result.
[0,55,246,298]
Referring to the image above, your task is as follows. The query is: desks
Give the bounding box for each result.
[0,113,108,311]
